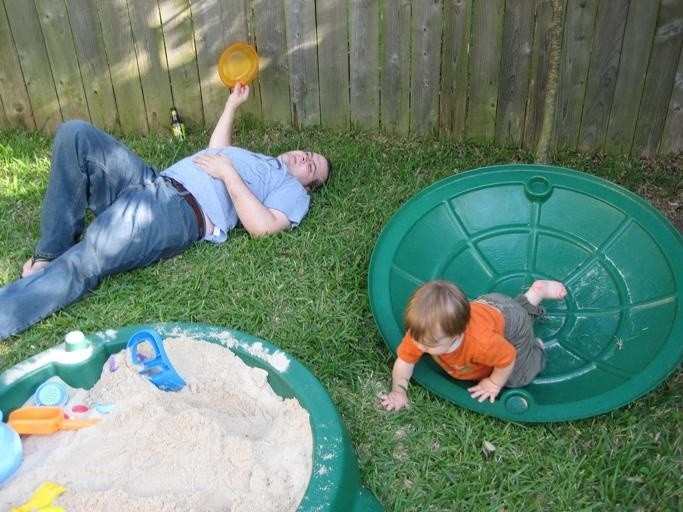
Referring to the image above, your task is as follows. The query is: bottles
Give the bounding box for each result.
[170,107,186,144]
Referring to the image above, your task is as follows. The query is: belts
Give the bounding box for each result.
[161,174,205,241]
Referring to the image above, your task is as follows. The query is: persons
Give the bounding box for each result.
[380,280,567,412]
[0,81,331,342]
